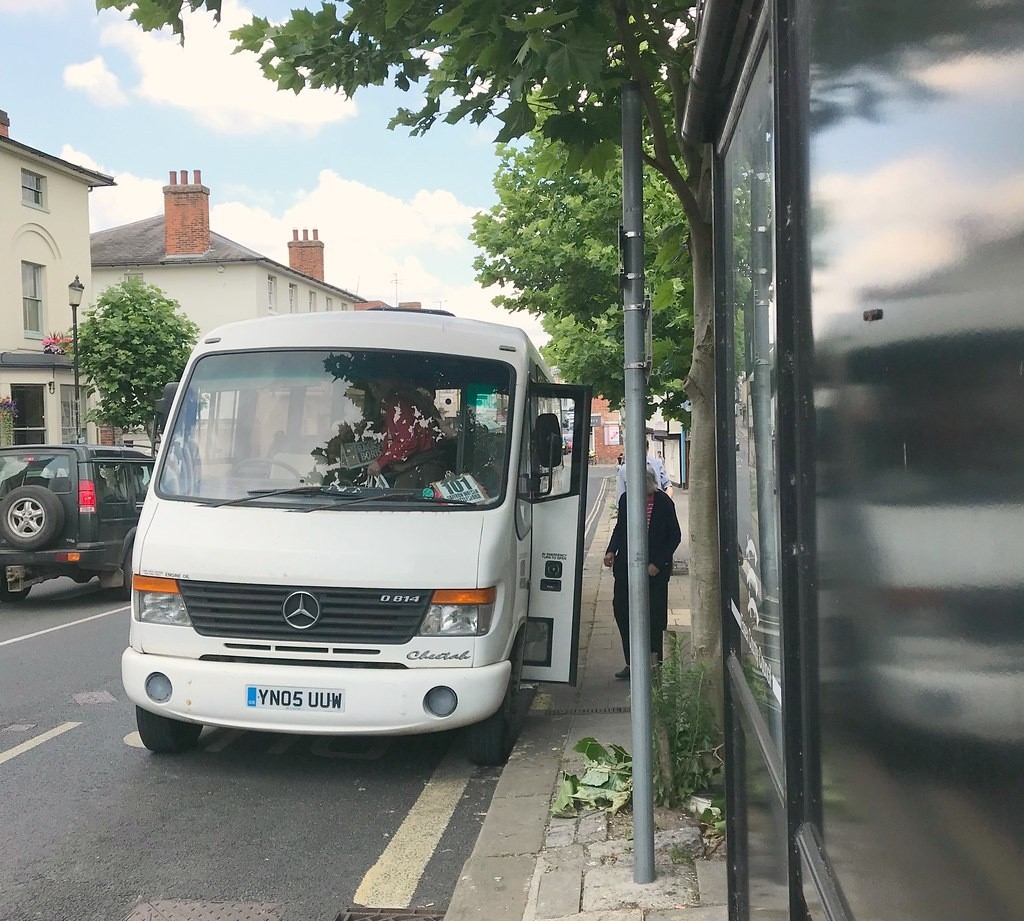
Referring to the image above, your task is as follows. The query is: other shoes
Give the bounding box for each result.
[615,666,630,678]
[652,662,663,678]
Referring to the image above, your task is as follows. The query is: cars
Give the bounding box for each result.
[561,435,573,455]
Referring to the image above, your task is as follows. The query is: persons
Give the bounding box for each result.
[604,465,681,678]
[614,452,623,466]
[616,440,673,509]
[656,451,664,464]
[366,374,491,490]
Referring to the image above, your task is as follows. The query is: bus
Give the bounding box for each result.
[122,308,593,764]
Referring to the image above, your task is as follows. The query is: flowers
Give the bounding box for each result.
[42,332,72,354]
[0,397,20,446]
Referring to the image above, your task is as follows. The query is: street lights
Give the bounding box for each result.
[68,275,84,443]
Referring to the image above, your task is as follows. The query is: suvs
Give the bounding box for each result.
[0,443,179,602]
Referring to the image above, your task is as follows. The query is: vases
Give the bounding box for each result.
[43,350,55,355]
